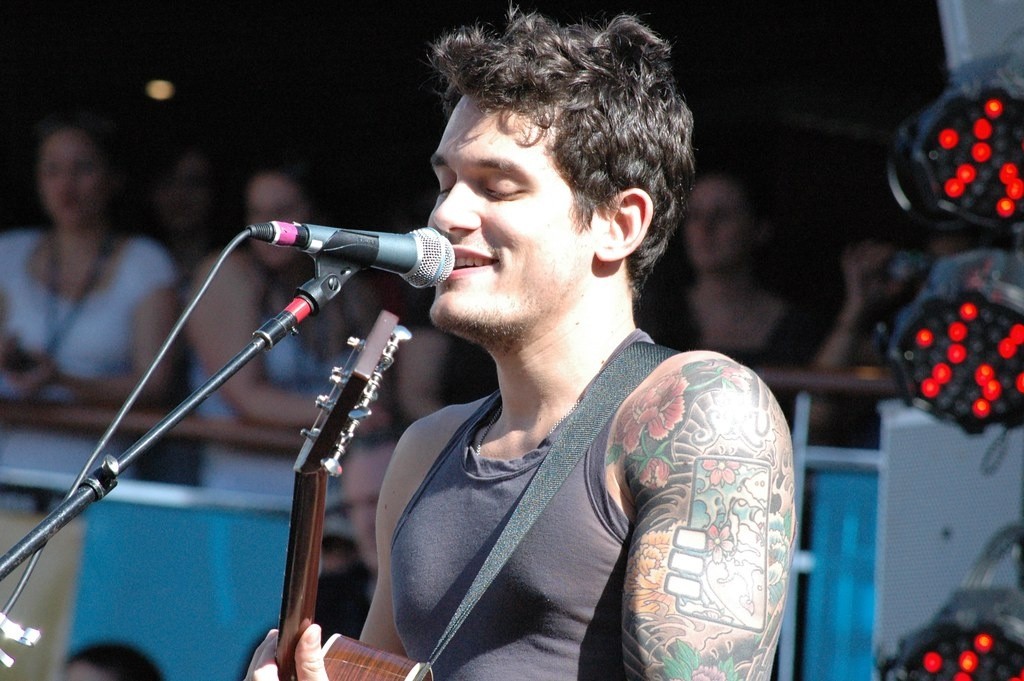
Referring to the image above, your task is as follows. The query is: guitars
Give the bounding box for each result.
[266,303,437,681]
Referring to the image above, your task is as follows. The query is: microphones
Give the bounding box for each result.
[248,220,457,288]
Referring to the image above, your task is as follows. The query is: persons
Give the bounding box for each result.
[0,127,498,647]
[244,1,795,681]
[62,643,164,681]
[651,178,965,447]
